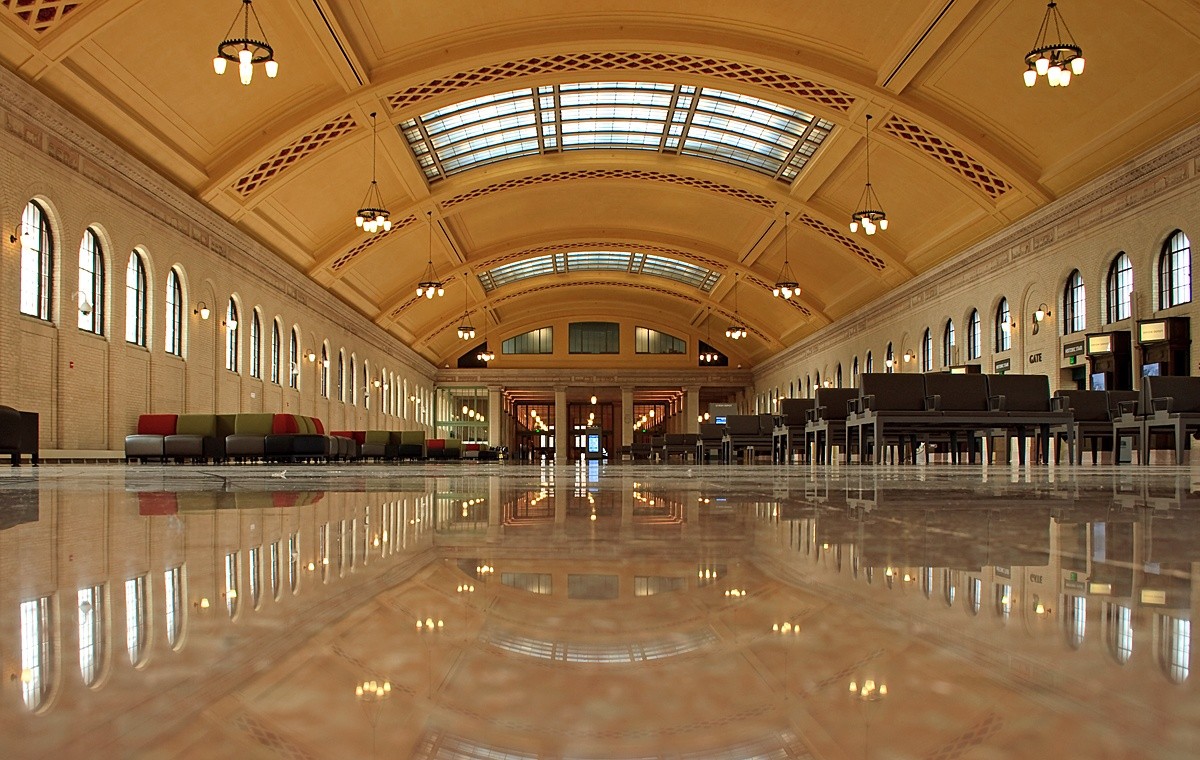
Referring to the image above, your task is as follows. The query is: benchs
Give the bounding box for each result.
[631,373,1200,466]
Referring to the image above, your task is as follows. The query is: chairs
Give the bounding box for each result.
[0,406,509,467]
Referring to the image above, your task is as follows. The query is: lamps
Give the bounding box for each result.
[382,382,389,390]
[355,113,393,233]
[320,358,329,367]
[726,273,748,340]
[372,377,379,387]
[477,304,495,362]
[904,349,915,362]
[885,352,897,367]
[303,349,315,361]
[457,272,476,341]
[213,0,279,86]
[779,393,786,400]
[223,318,237,330]
[850,115,888,237]
[194,301,209,320]
[823,377,833,387]
[773,211,802,300]
[408,392,415,401]
[814,382,821,390]
[10,223,32,249]
[1022,0,1086,90]
[1035,303,1051,321]
[1000,316,1016,332]
[415,210,444,299]
[700,306,719,363]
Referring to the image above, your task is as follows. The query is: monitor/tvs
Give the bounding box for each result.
[589,434,599,453]
[715,415,726,424]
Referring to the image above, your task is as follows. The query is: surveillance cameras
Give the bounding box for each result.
[79,301,91,315]
